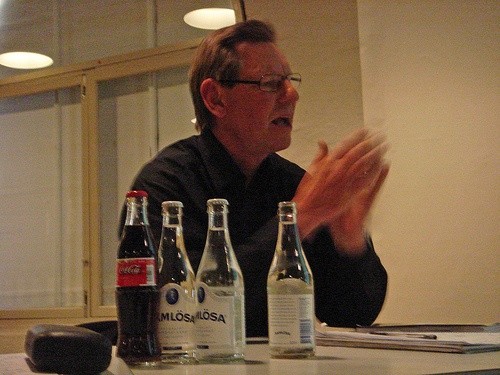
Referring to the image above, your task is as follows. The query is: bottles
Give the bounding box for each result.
[157,200,195,365]
[193,199,245,363]
[267,202,315,361]
[117,190,158,367]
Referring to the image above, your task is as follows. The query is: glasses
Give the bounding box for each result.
[222,72,303,93]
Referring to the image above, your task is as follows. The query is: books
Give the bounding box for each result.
[313,321,500,354]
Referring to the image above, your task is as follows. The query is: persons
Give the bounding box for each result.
[115,20,392,337]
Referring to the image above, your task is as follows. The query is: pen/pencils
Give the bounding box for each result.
[369,330,438,341]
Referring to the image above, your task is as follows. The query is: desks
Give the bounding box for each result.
[135,330,500,375]
